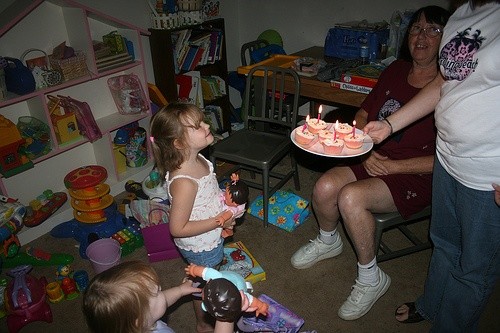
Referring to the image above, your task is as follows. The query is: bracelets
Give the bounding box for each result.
[382,118,393,134]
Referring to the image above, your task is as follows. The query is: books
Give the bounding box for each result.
[172,30,225,136]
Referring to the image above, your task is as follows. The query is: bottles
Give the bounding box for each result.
[360,40,369,65]
[150,172,159,187]
[390,10,415,57]
[381,45,386,60]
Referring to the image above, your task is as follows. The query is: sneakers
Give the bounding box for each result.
[338,265,391,321]
[291,231,344,270]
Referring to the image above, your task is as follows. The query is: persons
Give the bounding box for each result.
[82,103,259,333]
[184,263,271,317]
[290,4,453,321]
[360,0,500,333]
[215,174,249,238]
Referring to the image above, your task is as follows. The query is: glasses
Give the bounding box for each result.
[407,23,442,39]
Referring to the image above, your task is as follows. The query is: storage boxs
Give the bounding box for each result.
[342,64,385,86]
[140,224,180,262]
[218,241,267,285]
[330,80,373,96]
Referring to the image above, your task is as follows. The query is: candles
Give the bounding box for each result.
[318,104,322,122]
[352,120,356,136]
[303,114,310,131]
[334,120,338,140]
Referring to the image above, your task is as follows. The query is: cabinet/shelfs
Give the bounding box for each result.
[146,18,232,134]
[0,0,159,248]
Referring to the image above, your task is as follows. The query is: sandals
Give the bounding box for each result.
[395,302,425,323]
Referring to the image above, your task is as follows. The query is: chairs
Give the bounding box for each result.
[206,66,302,228]
[240,39,316,123]
[373,208,431,262]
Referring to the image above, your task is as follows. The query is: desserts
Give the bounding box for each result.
[295,118,364,155]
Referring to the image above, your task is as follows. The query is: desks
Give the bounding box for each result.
[254,46,372,119]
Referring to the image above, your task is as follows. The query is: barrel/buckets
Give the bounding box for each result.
[86,238,121,275]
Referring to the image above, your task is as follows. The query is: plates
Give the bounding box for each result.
[290,123,373,158]
